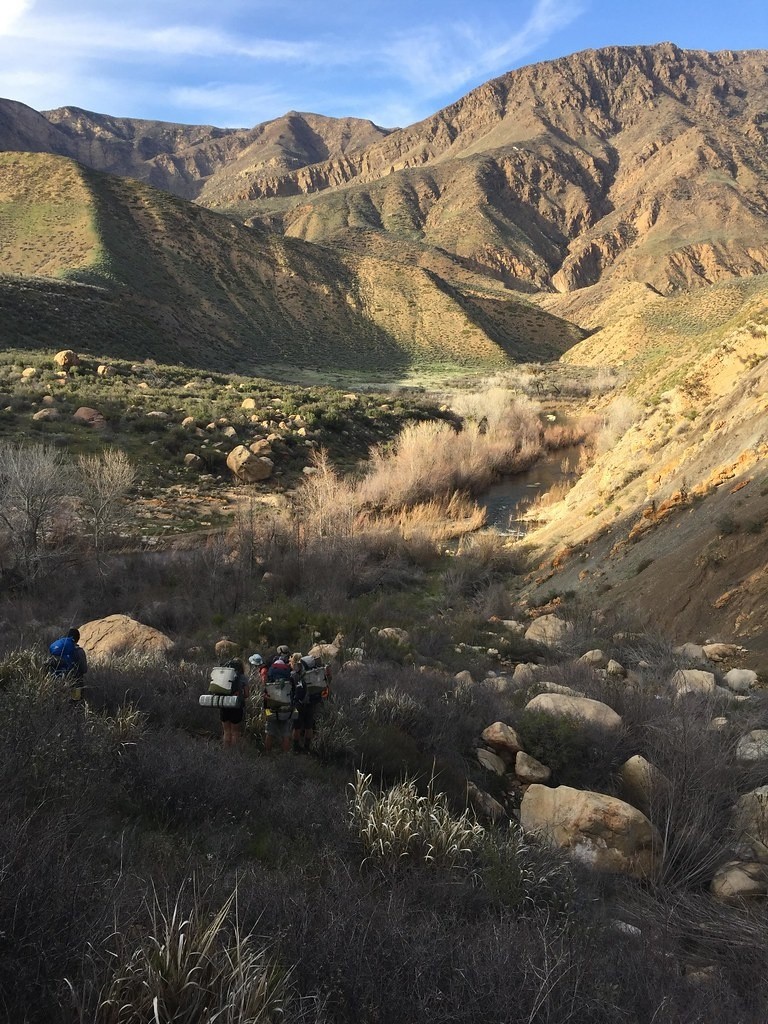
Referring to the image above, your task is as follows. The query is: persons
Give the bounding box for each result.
[44,628,88,700]
[216,658,250,742]
[249,653,332,751]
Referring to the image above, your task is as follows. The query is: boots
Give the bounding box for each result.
[291,738,299,754]
[302,737,311,754]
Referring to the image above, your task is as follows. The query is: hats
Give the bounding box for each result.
[249,654,263,665]
[277,645,293,658]
[68,628,80,643]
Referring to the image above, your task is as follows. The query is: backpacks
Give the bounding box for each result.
[290,655,332,706]
[41,638,83,682]
[264,656,299,721]
[207,660,244,696]
